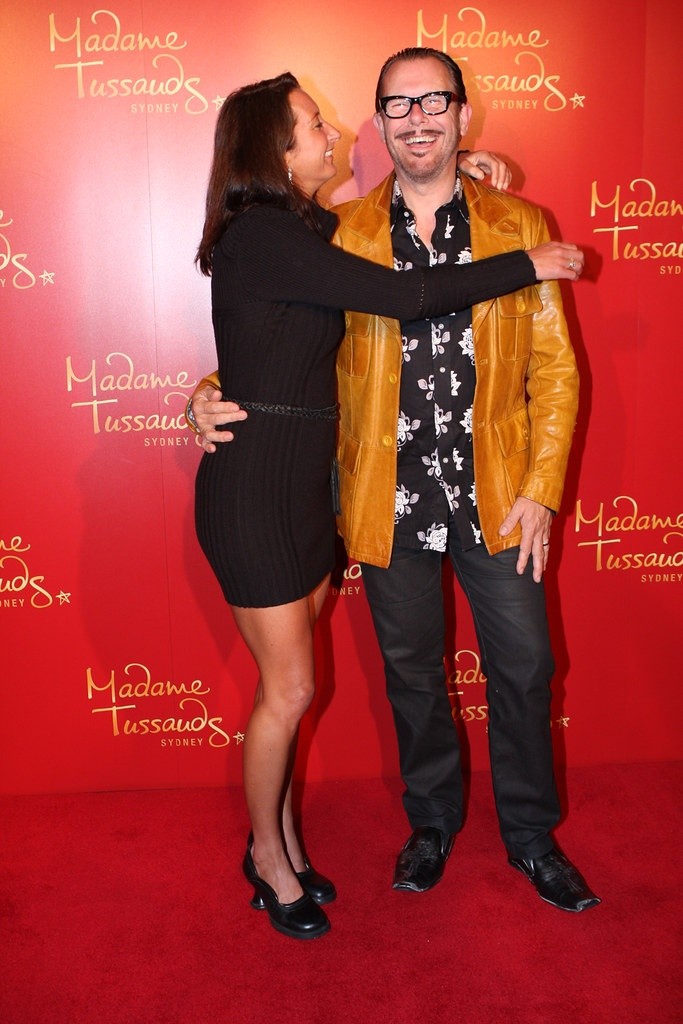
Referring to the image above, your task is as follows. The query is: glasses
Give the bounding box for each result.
[378,91,460,119]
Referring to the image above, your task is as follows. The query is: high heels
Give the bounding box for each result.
[244,844,331,939]
[281,846,337,904]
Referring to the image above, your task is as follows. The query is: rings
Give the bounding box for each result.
[569,258,577,269]
[543,542,550,547]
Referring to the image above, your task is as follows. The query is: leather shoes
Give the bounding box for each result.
[391,825,457,891]
[507,836,603,912]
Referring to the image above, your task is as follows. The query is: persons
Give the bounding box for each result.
[192,76,583,942]
[183,47,601,913]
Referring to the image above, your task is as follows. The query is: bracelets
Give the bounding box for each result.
[188,404,200,434]
